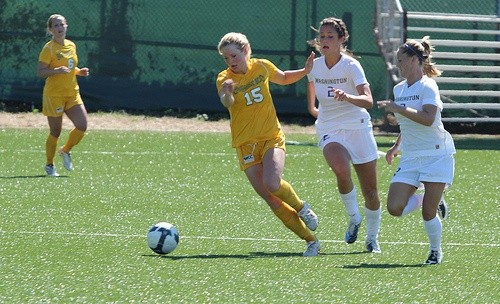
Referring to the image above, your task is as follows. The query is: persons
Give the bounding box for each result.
[216,32,320,257]
[37,14,89,177]
[306,17,382,254]
[377,36,456,264]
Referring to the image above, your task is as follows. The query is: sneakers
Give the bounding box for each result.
[45,163,59,177]
[426,249,442,264]
[366,238,381,253]
[297,201,318,231]
[437,195,448,220]
[303,235,320,256]
[345,217,362,243]
[59,146,74,171]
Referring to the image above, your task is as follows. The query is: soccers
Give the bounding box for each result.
[146,222,179,255]
